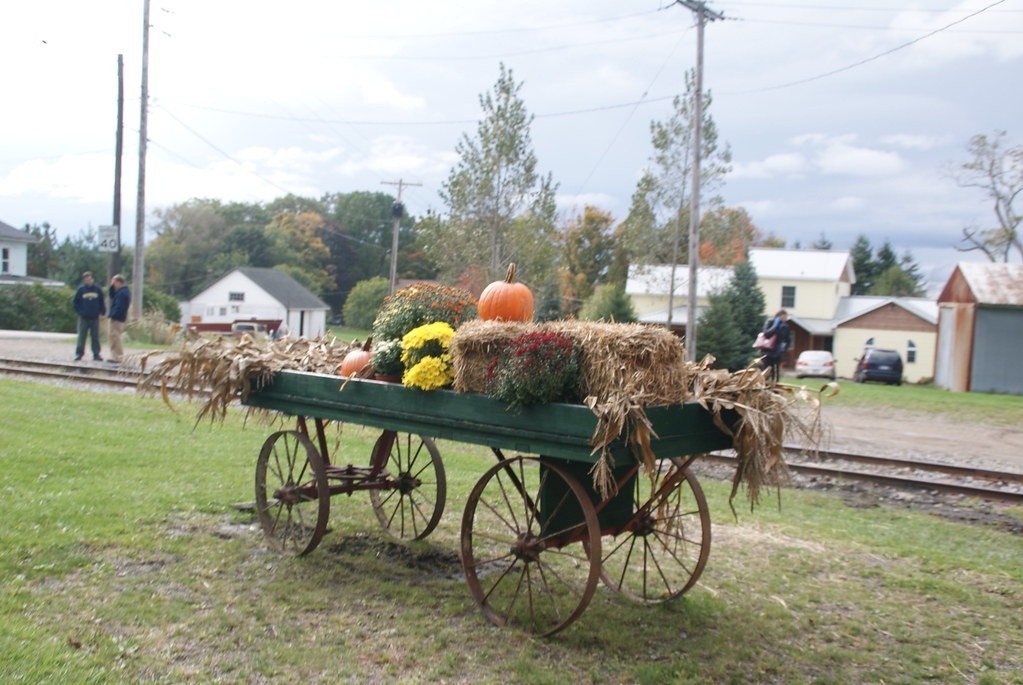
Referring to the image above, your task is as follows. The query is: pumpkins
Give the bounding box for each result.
[477,262,533,322]
[340,335,376,380]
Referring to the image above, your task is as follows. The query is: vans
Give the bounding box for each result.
[233,322,263,335]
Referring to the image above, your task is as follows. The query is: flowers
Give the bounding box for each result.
[370,282,582,418]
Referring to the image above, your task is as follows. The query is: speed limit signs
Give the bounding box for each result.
[98,225,118,253]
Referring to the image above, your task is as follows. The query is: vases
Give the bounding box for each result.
[374,373,402,384]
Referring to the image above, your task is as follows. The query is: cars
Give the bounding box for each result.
[330,314,344,326]
[853,348,904,385]
[793,350,837,381]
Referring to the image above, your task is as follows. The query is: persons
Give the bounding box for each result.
[107,274,131,363]
[760,310,791,382]
[72,270,106,362]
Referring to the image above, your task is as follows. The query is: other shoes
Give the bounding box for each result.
[95,355,103,361]
[75,356,81,361]
[107,359,123,363]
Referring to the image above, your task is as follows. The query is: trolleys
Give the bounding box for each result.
[239,366,744,640]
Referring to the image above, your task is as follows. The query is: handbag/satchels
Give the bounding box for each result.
[752,332,778,350]
